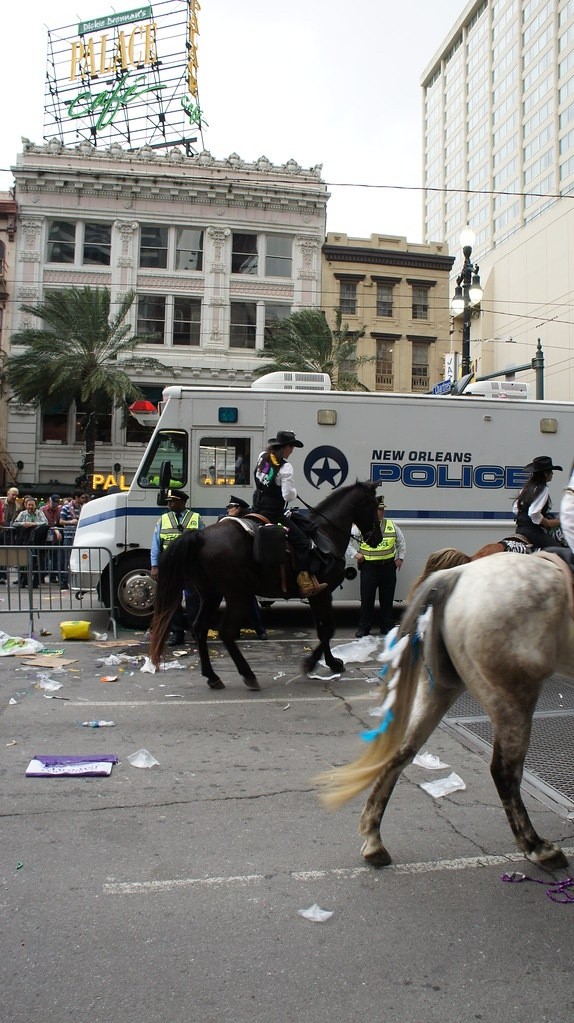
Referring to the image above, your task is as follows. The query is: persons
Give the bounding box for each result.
[251,430,327,596]
[512,455,563,550]
[150,488,205,647]
[218,495,270,640]
[346,495,405,638]
[0,486,90,591]
[558,458,574,577]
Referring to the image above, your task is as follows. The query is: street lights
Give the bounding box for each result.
[450,219,485,395]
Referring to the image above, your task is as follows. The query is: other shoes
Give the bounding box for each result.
[168,635,185,646]
[257,628,269,640]
[380,628,390,635]
[0,573,68,590]
[355,629,370,638]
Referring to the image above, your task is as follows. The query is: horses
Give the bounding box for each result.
[308,531,574,868]
[147,479,383,690]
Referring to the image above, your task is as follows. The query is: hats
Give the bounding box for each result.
[226,494,250,510]
[375,496,387,507]
[523,456,563,473]
[267,430,303,448]
[164,489,189,502]
[51,494,60,503]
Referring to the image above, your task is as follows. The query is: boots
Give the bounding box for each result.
[296,571,328,594]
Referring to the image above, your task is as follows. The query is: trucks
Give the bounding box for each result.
[64,366,574,630]
